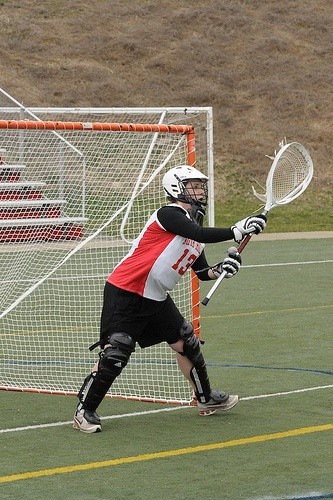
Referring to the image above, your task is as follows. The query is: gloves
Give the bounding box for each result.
[231,213,269,243]
[211,246,242,281]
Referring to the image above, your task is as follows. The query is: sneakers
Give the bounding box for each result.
[196,391,239,417]
[72,406,103,433]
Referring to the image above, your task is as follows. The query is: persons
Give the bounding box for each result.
[72,165,267,433]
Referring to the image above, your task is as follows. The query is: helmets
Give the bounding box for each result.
[162,164,210,227]
